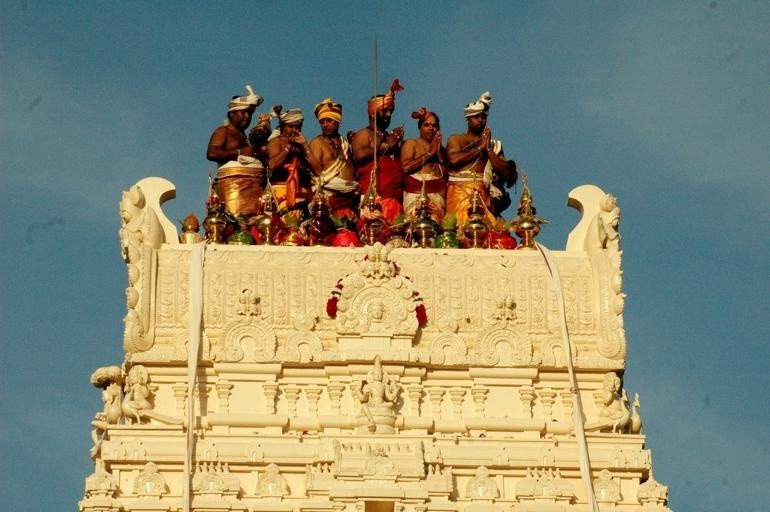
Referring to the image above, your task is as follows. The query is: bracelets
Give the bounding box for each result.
[284,146,290,152]
[426,151,432,158]
[304,148,311,154]
[381,143,389,151]
[486,147,492,152]
[476,146,483,151]
[236,148,241,156]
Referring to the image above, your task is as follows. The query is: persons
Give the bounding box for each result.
[207,85,265,194]
[265,107,321,194]
[305,97,357,195]
[352,367,404,412]
[369,301,386,321]
[350,78,407,212]
[400,110,448,219]
[446,89,509,231]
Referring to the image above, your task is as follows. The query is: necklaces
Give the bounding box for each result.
[325,133,342,156]
[366,125,387,142]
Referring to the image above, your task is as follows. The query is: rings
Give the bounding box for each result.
[483,133,486,136]
